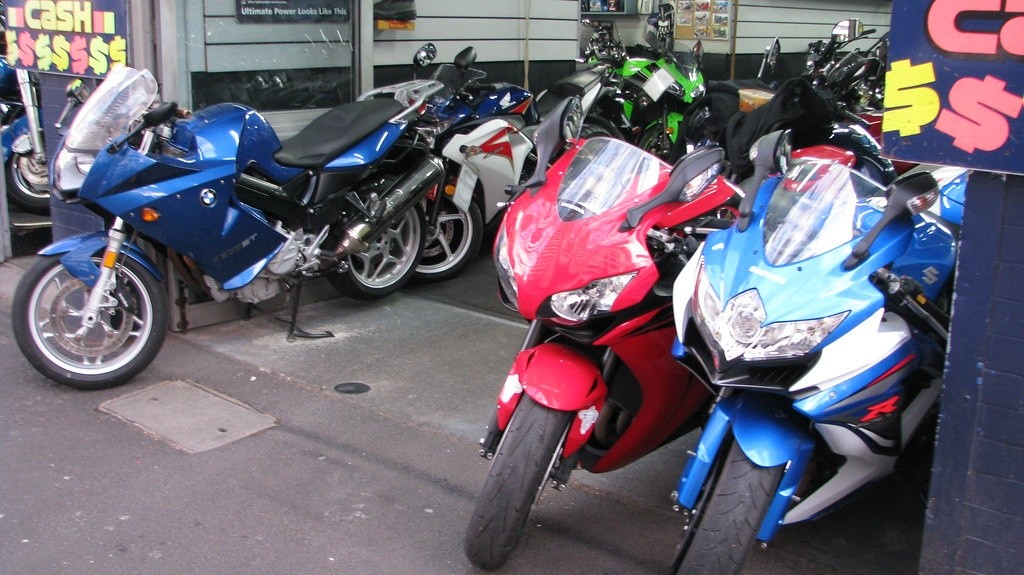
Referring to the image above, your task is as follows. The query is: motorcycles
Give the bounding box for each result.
[0,17,69,212]
[12,65,444,391]
[463,93,973,575]
[412,4,921,284]
